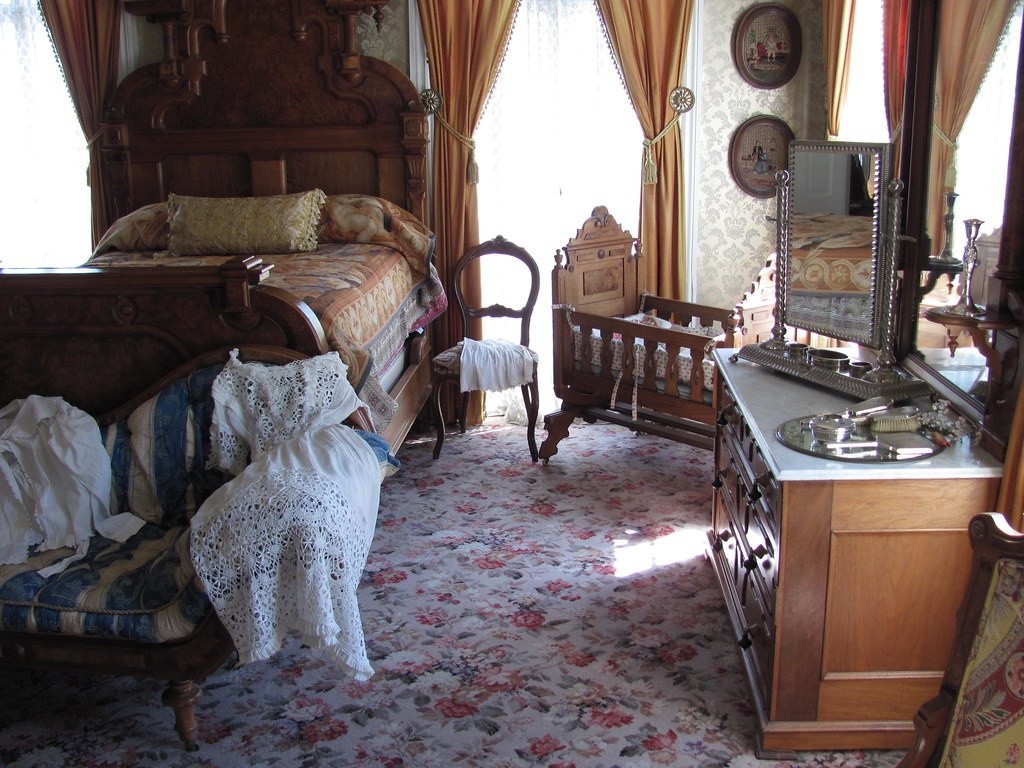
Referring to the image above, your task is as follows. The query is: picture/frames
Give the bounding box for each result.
[730,115,796,199]
[733,4,802,91]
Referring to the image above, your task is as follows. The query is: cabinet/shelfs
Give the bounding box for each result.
[705,348,1003,752]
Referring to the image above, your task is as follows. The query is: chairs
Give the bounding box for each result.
[430,235,539,461]
[0,349,401,753]
[896,511,1024,768]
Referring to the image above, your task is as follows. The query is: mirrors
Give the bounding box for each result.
[783,138,894,347]
[903,0,1024,410]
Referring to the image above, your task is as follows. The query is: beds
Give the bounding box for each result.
[0,53,450,457]
[539,206,819,465]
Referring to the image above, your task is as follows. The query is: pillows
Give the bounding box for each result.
[166,188,328,254]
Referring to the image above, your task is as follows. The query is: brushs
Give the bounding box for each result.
[869,406,921,433]
[847,395,894,425]
